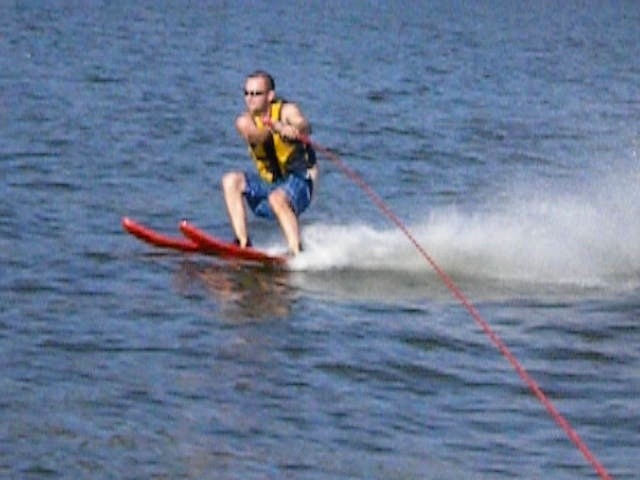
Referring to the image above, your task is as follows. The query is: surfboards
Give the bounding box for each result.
[178,221,284,264]
[119,216,198,250]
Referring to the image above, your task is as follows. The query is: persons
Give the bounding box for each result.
[221,70,316,259]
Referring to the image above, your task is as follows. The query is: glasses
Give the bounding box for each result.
[244,90,264,97]
